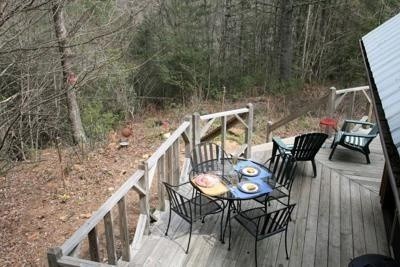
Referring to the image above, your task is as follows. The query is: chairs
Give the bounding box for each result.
[161,118,380,267]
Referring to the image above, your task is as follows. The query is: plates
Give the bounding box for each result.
[239,165,262,178]
[194,173,220,187]
[236,180,263,194]
